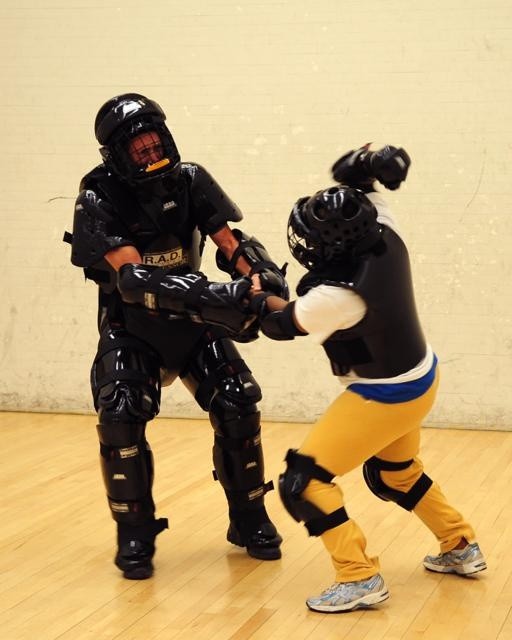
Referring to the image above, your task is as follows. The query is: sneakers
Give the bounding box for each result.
[114,550,155,579]
[306,574,389,615]
[226,526,282,561]
[422,541,488,577]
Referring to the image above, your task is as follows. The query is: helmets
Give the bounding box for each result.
[94,93,181,186]
[286,185,378,274]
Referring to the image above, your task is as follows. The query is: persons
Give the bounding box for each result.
[70,94,289,581]
[241,140,488,615]
